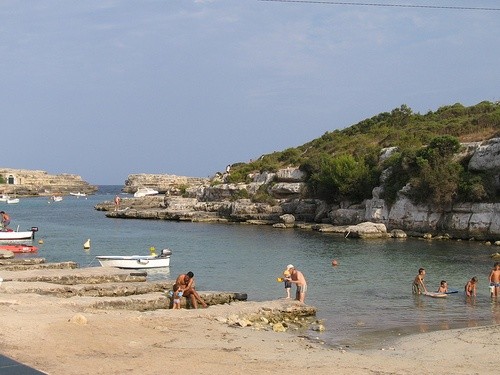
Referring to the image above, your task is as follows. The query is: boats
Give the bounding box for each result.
[0,194,8,201]
[51,196,63,202]
[95,249,171,272]
[133,188,158,197]
[69,192,86,197]
[7,198,19,203]
[1,225,39,246]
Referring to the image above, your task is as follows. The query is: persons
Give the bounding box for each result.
[281,270,293,299]
[286,264,307,302]
[226,164,231,173]
[437,280,449,293]
[172,284,183,309]
[176,272,208,309]
[488,262,500,298]
[114,194,121,211]
[464,276,478,297]
[0,211,11,231]
[411,268,428,295]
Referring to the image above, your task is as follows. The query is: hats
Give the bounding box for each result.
[284,264,294,275]
